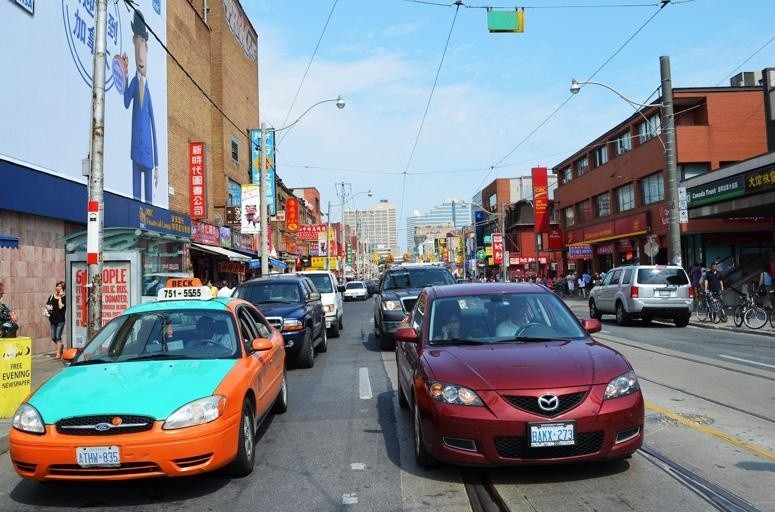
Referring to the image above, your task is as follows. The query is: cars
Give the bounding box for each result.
[8,275,297,487]
[282,269,346,341]
[393,278,647,471]
[343,277,381,299]
[140,271,195,303]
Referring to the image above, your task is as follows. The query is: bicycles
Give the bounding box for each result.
[697,287,775,331]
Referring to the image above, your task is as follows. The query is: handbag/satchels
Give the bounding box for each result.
[43,308,52,318]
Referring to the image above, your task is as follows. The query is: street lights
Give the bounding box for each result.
[259,91,349,276]
[460,200,507,282]
[569,74,684,266]
[325,188,375,271]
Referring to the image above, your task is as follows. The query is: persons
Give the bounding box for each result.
[757,262,773,311]
[434,313,472,340]
[495,298,533,337]
[185,315,232,350]
[206,279,246,298]
[120,9,160,204]
[45,280,66,360]
[677,256,726,319]
[0,281,19,337]
[476,269,606,299]
[282,286,298,301]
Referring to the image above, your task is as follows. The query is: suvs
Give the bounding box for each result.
[373,263,470,345]
[587,264,696,330]
[224,272,330,371]
